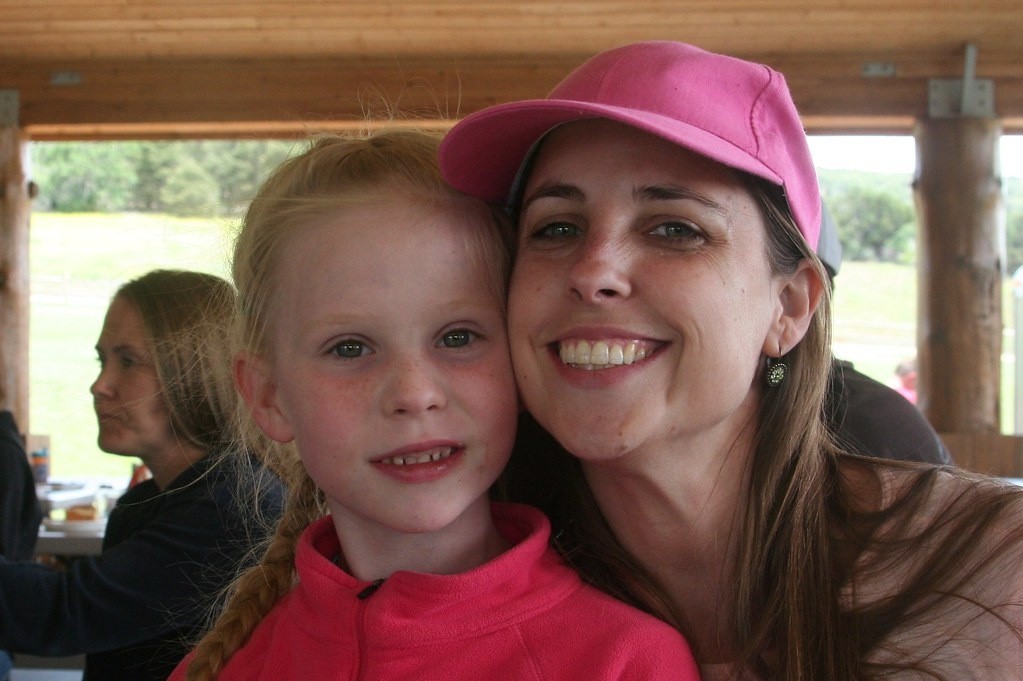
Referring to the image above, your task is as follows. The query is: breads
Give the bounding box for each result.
[66,504,96,520]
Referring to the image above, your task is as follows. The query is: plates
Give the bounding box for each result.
[45,488,101,510]
[41,519,110,533]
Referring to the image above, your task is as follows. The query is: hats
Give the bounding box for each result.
[437,41,821,254]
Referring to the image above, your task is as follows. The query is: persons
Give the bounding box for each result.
[81,269,296,681]
[435,41,1023,681]
[167,129,702,681]
[1,409,44,680]
